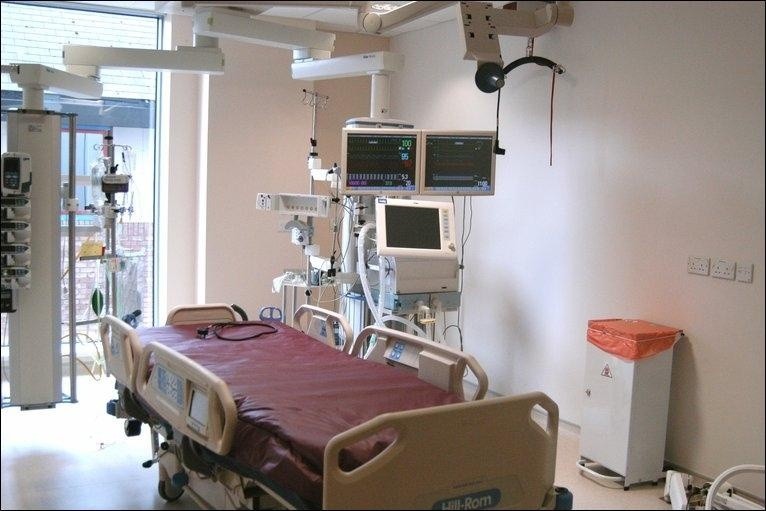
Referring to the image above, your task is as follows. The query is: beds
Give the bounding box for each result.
[99,304,574,510]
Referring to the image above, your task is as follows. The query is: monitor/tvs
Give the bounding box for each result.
[375,198,454,257]
[342,128,419,195]
[421,130,496,196]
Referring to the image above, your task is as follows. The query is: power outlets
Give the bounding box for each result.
[685,255,754,284]
